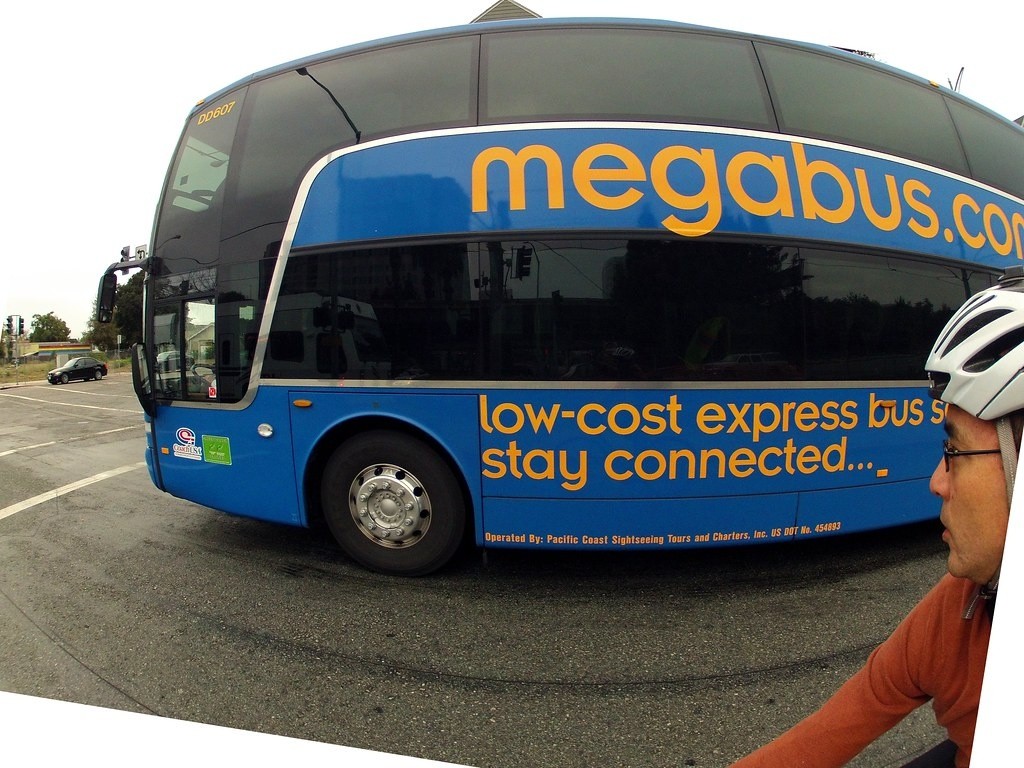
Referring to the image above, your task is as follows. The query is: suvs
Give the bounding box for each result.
[701,351,784,383]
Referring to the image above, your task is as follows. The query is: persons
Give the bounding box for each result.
[104,361,108,375]
[724,282,1024,768]
[190,358,198,375]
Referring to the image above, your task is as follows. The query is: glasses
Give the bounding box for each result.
[943,439,1001,472]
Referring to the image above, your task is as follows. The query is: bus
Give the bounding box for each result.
[95,13,1024,585]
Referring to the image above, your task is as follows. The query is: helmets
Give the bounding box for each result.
[925,265,1024,420]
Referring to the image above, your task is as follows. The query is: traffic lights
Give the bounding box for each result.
[19,318,24,336]
[6,316,12,334]
[515,247,533,277]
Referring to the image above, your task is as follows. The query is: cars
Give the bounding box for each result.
[46,356,108,385]
[153,351,195,374]
